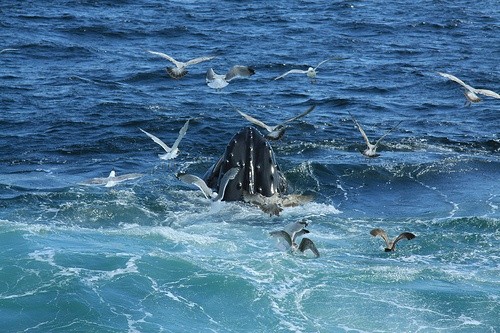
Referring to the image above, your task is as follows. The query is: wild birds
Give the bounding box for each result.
[434,70,500,103]
[232,104,317,142]
[137,118,190,161]
[171,165,242,203]
[243,190,286,218]
[204,64,256,92]
[268,218,322,258]
[75,170,143,188]
[274,56,343,81]
[370,227,416,253]
[346,109,404,159]
[145,49,219,80]
